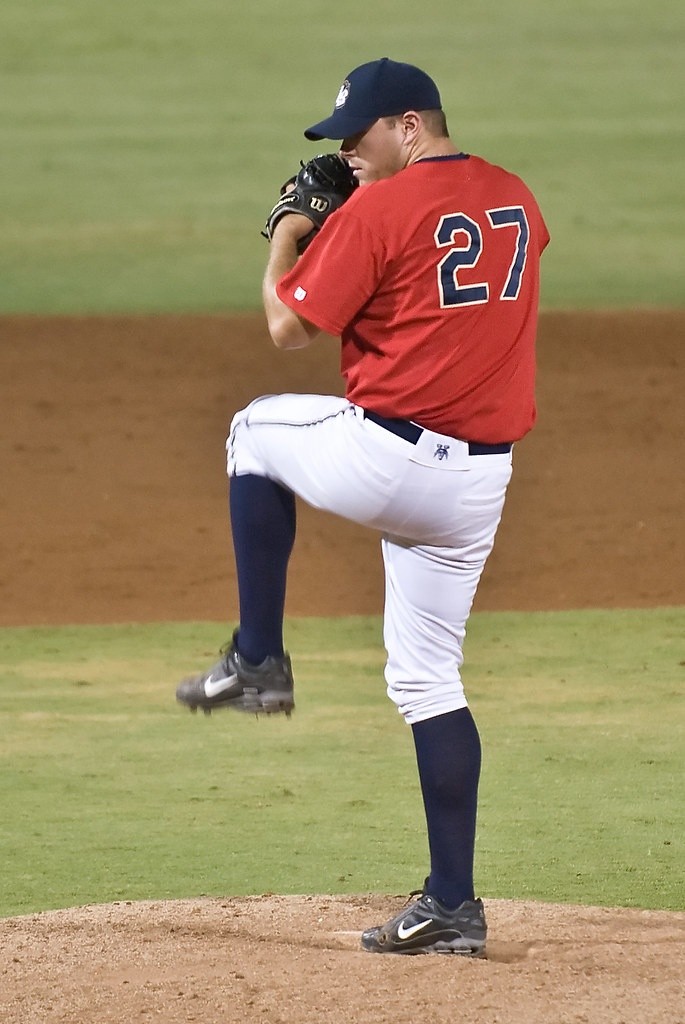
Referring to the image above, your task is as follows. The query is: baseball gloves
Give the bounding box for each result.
[265,152,360,256]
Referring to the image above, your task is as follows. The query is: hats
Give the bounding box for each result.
[304,58,441,141]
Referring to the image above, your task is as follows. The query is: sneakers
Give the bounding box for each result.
[359,877,486,956]
[174,628,295,714]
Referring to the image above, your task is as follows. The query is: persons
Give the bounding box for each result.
[176,57,552,962]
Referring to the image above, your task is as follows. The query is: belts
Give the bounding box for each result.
[364,409,511,456]
